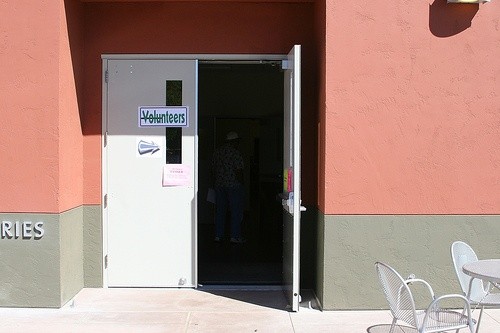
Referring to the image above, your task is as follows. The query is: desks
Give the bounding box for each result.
[462,258,500,333]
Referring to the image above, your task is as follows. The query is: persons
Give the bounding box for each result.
[208,130,246,244]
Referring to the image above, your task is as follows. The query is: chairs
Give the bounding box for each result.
[450,241,500,333]
[374,262,477,332]
